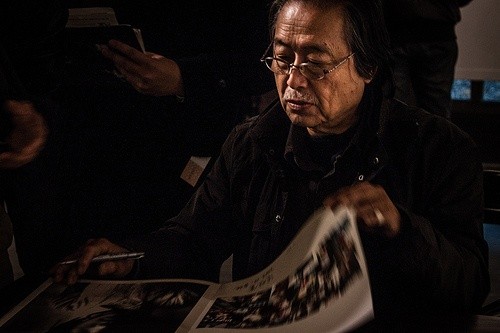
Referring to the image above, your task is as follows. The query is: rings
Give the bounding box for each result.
[374,208,388,227]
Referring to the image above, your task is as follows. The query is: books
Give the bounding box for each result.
[0,194,373,333]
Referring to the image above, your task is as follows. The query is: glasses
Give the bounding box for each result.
[259,41,356,81]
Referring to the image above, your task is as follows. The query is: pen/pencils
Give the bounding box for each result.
[59,251,147,266]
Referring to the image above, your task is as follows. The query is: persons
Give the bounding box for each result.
[50,280,196,333]
[0,1,274,275]
[381,0,471,119]
[45,1,492,333]
